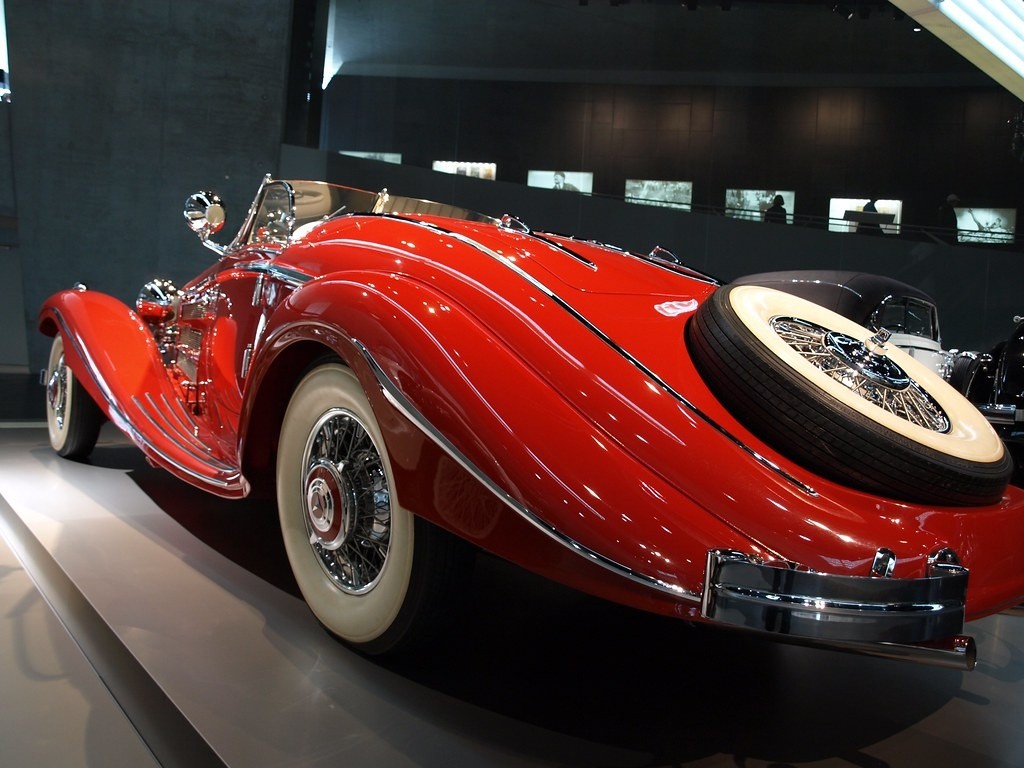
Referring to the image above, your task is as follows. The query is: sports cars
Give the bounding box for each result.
[38,172,1024,662]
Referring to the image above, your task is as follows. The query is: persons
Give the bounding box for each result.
[765,195,786,224]
[857,199,883,234]
[553,172,579,191]
[968,208,1001,231]
